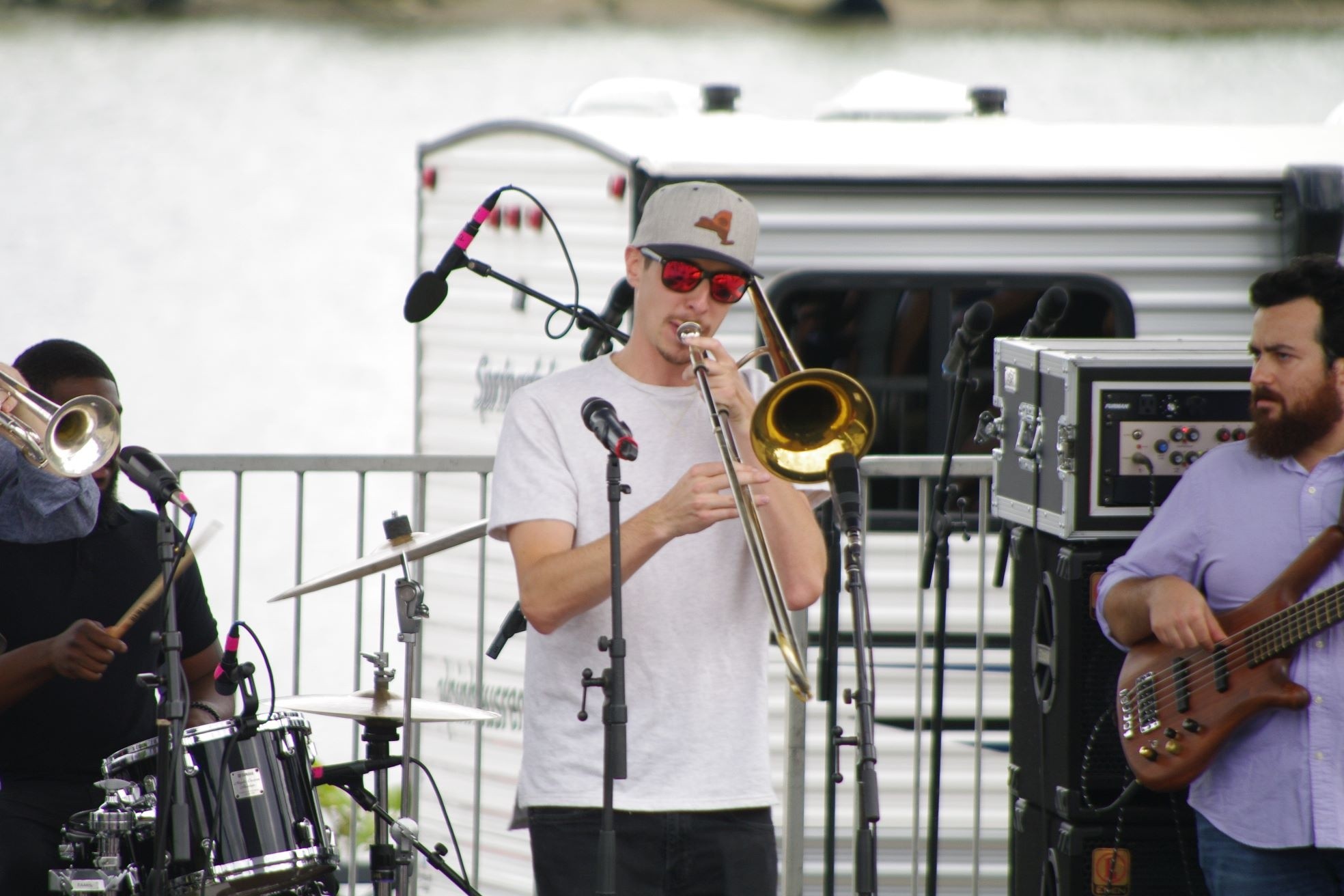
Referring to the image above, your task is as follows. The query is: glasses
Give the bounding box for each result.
[641,247,751,304]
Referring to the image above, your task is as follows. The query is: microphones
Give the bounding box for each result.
[116,446,196,517]
[311,756,413,786]
[403,189,501,323]
[581,278,634,363]
[1021,287,1068,337]
[214,625,239,696]
[942,301,994,378]
[582,397,638,463]
[828,453,864,558]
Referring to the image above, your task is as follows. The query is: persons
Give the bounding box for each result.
[0,340,237,896]
[486,183,833,896]
[1096,260,1344,896]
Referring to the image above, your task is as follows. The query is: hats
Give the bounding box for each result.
[630,178,766,281]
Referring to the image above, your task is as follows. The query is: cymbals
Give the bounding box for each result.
[258,690,503,723]
[266,519,489,603]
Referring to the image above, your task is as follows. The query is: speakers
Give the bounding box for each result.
[1005,525,1211,896]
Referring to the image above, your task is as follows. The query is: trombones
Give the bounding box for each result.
[673,277,879,703]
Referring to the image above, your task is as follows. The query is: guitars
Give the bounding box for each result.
[1115,523,1344,795]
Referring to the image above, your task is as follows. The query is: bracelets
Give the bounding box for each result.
[190,701,222,722]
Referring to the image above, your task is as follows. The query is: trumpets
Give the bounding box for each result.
[0,372,123,480]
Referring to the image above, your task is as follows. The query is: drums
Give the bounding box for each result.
[101,711,341,895]
[64,801,156,896]
